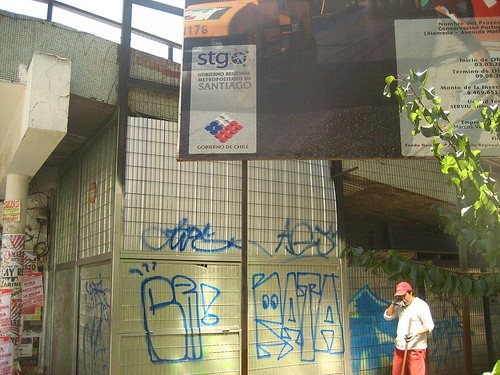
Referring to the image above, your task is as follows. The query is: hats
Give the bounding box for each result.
[394,282,413,296]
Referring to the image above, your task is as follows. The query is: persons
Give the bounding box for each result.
[383,282,435,375]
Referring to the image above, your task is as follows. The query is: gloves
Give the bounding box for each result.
[404,332,418,342]
[392,298,408,309]
